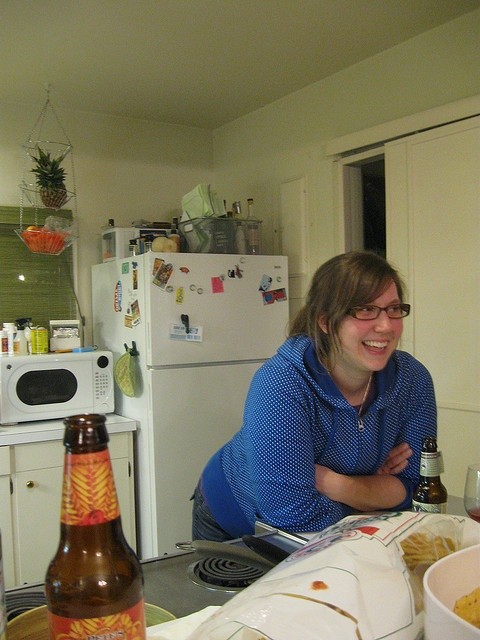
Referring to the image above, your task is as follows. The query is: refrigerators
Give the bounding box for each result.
[91,251,289,561]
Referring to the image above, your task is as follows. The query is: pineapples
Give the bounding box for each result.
[29,145,69,207]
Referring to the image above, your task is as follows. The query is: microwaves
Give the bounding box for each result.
[0,351,115,425]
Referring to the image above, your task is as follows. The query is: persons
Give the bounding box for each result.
[190,252,437,544]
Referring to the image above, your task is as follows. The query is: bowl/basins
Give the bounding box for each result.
[421,541,480,640]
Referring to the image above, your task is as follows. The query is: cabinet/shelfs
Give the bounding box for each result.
[0,431,136,589]
[13,101,79,256]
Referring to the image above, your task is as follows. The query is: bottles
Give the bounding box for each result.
[0,322,17,353]
[246,198,262,254]
[30,327,49,354]
[233,200,246,254]
[45,413,147,640]
[129,233,155,256]
[13,317,32,353]
[412,437,448,515]
[168,215,186,253]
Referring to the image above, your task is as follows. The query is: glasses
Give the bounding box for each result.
[346,304,410,320]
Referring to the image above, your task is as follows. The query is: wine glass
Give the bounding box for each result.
[463,464,480,523]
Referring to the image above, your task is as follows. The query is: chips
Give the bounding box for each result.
[401,533,457,613]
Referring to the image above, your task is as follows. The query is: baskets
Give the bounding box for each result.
[12,226,80,256]
[23,140,72,162]
[21,184,75,210]
